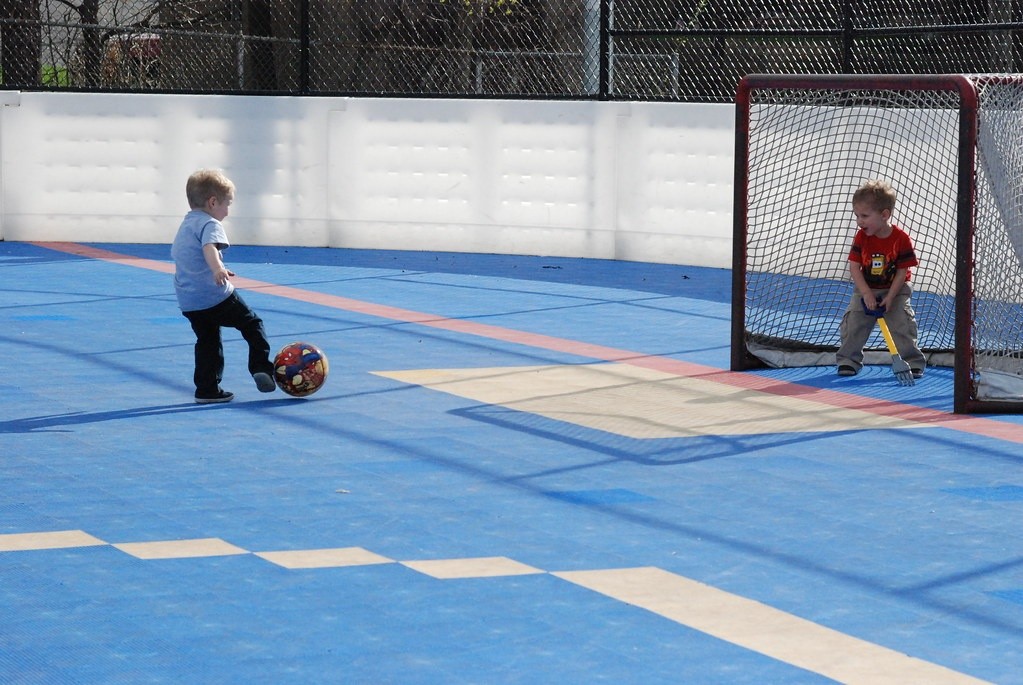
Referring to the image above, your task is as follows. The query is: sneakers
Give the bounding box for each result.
[252,371,276,393]
[909,368,923,377]
[195,387,234,403]
[837,365,856,375]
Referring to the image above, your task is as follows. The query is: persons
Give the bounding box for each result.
[835,178,928,379]
[170,168,278,403]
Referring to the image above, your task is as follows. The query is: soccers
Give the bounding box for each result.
[272,341,330,398]
